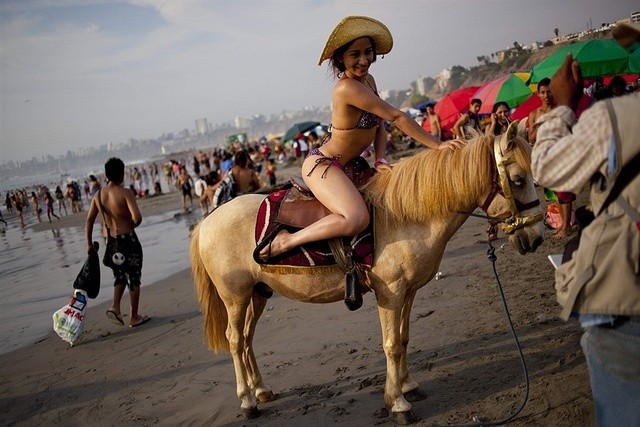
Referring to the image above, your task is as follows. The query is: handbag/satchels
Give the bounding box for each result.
[543,201,576,229]
[562,236,578,264]
[102,235,133,271]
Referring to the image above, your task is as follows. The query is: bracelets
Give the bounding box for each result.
[374,157,388,165]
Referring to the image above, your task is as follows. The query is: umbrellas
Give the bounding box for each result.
[524,38,640,87]
[283,121,320,143]
[468,71,540,115]
[422,87,482,132]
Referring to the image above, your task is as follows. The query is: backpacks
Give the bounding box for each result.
[213,169,238,209]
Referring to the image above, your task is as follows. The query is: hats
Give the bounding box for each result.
[318,16,393,65]
[612,21,640,49]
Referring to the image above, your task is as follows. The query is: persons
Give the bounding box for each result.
[527,77,557,145]
[85,157,151,327]
[260,136,286,159]
[266,159,278,186]
[484,101,511,135]
[292,132,308,167]
[453,98,482,140]
[529,19,640,427]
[1,181,84,225]
[426,103,443,142]
[551,192,577,239]
[608,75,627,96]
[308,131,322,159]
[258,17,469,263]
[85,169,108,200]
[125,164,151,198]
[151,130,258,214]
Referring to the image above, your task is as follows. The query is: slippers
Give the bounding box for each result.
[129,314,151,328]
[253,224,291,264]
[106,310,124,326]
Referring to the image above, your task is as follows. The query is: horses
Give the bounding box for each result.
[190,119,546,425]
